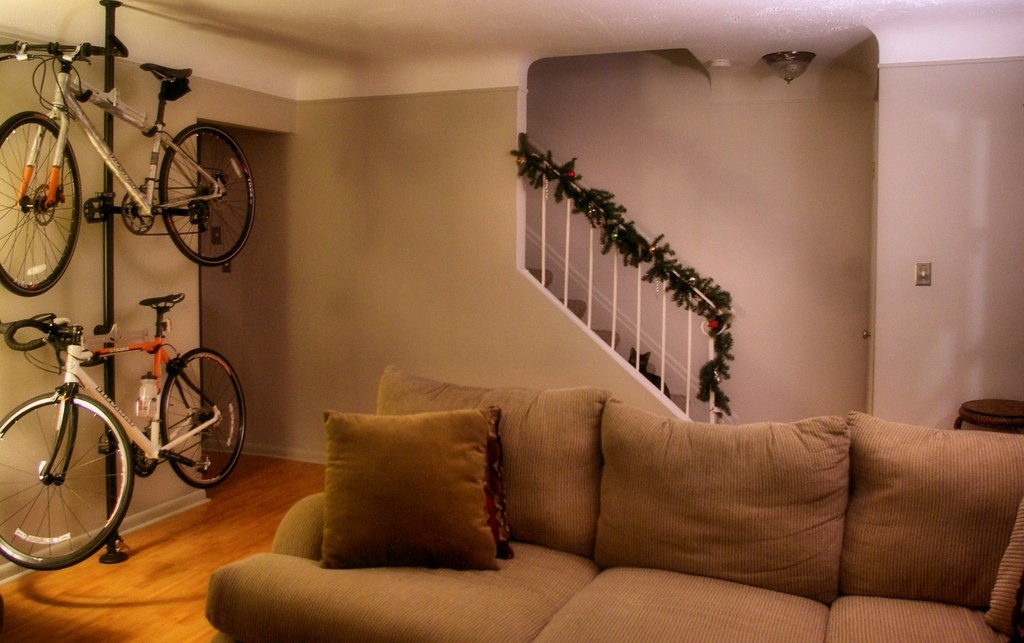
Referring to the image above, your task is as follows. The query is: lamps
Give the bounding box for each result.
[762,51,816,85]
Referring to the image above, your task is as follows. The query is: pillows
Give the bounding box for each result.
[483,407,514,560]
[984,497,1024,643]
[377,364,613,558]
[837,408,1024,609]
[595,397,848,603]
[317,409,500,571]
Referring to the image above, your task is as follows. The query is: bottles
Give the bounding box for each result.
[137,372,159,417]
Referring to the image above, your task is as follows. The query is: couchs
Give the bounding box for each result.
[207,492,1024,643]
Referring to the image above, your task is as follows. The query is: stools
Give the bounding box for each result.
[954,398,1024,435]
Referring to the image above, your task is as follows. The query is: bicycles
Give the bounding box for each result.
[0,33,256,298]
[0,292,248,570]
